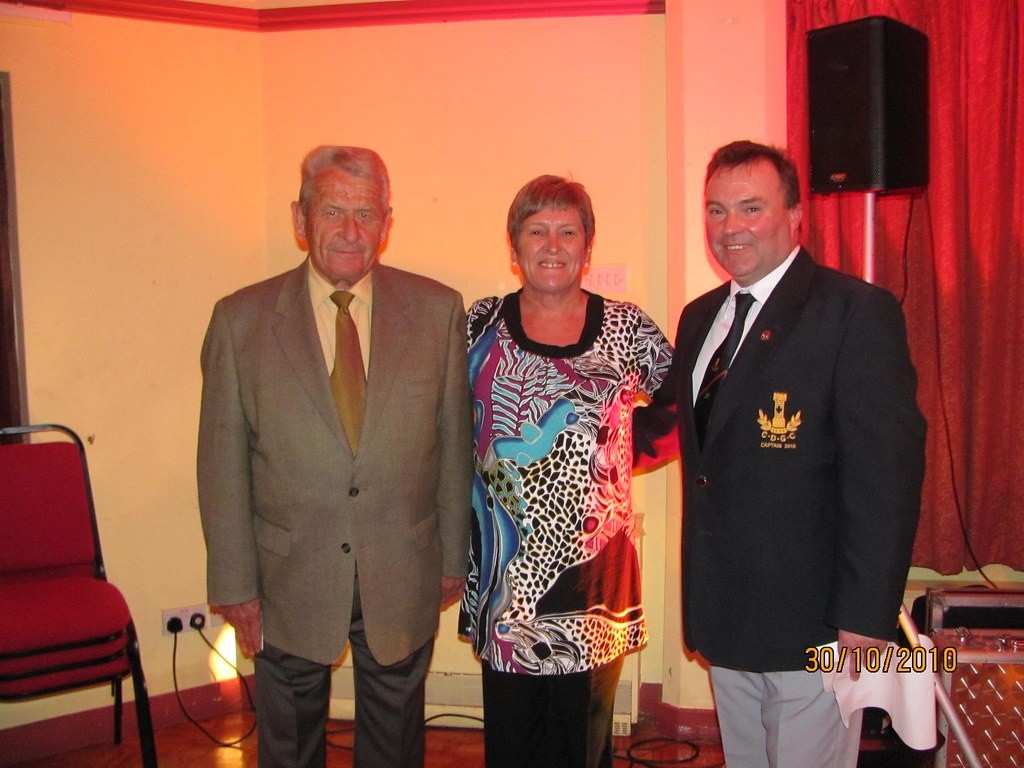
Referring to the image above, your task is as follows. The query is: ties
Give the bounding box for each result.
[692,292,757,457]
[326,291,366,457]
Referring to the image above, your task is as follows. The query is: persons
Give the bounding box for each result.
[632,139,927,768]
[197,147,473,768]
[457,174,681,768]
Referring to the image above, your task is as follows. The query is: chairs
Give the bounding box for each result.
[0,422,160,768]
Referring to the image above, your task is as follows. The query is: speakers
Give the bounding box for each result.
[805,15,929,196]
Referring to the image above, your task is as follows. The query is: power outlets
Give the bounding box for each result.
[162,604,212,633]
